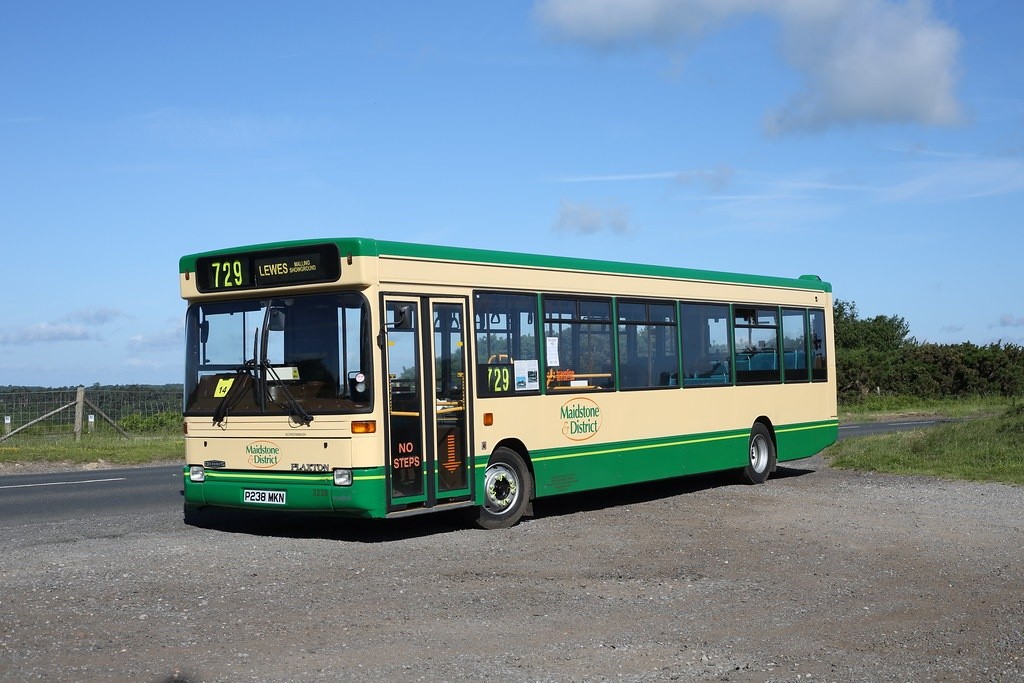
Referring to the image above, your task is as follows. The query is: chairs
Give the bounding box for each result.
[601,345,816,386]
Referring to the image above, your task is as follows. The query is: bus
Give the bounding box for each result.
[181,236,842,532]
